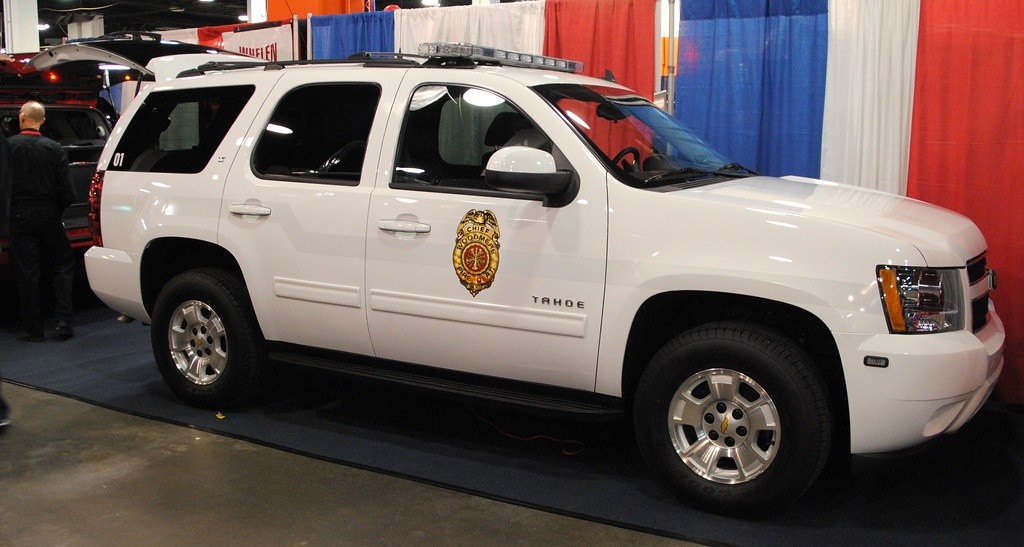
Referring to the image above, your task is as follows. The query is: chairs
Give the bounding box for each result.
[478,112,553,189]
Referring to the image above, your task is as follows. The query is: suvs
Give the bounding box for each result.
[0,31,162,293]
[23,40,1007,521]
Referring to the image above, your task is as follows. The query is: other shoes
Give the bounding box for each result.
[0,405,12,426]
[15,328,45,342]
[57,319,74,339]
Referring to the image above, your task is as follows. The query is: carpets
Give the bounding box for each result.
[0,299,1024,547]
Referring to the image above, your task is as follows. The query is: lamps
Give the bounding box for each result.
[36,15,50,31]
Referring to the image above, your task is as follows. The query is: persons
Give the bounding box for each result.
[0,101,72,341]
